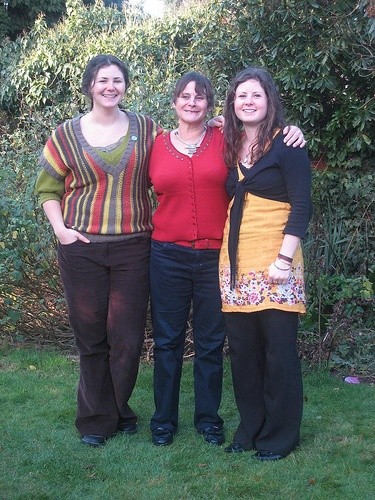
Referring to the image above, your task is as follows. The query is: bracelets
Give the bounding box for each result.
[278,253,293,265]
[274,260,291,271]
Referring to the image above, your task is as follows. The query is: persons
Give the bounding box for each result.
[35,54,224,446]
[218,69,312,460]
[148,72,306,445]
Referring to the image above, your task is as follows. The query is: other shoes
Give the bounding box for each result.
[224,441,247,453]
[119,422,137,435]
[150,425,174,446]
[202,425,225,446]
[81,435,107,448]
[250,450,282,461]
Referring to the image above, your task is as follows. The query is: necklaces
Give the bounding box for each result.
[174,125,206,153]
[241,137,252,162]
